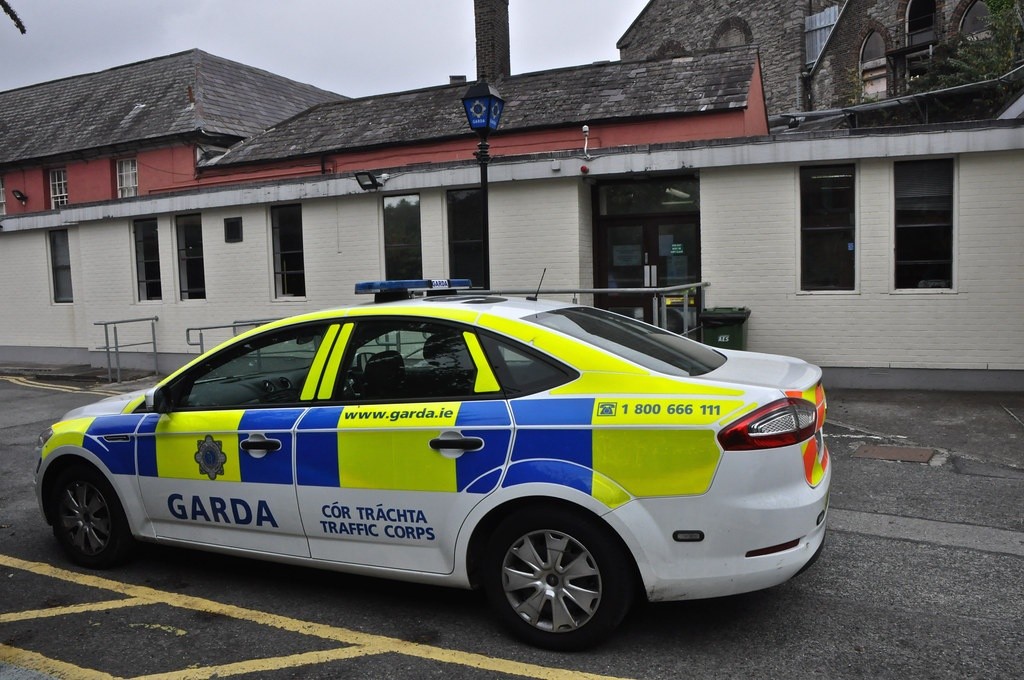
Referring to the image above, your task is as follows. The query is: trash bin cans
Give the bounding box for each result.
[699,306,752,350]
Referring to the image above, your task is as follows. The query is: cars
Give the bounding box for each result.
[33,293,832,652]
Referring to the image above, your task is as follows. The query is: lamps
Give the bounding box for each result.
[11,189,27,201]
[354,171,389,190]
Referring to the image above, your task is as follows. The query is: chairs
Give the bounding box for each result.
[361,350,405,399]
[423,335,468,394]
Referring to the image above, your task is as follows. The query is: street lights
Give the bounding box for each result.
[460,65,505,289]
